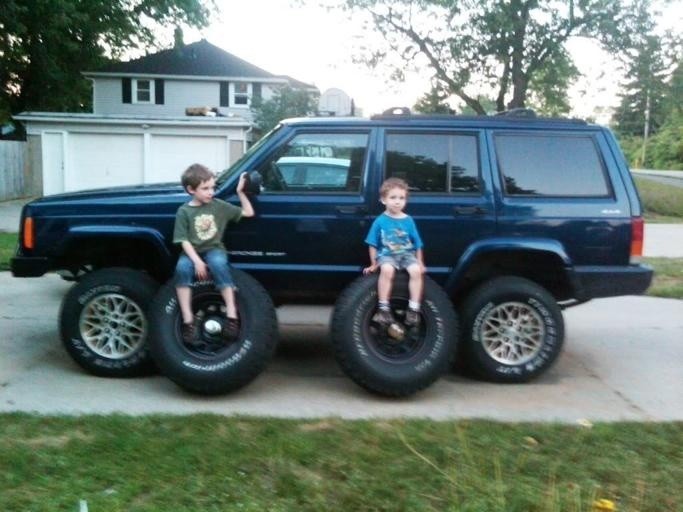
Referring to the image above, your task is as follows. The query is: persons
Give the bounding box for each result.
[173,162,254,345]
[362,175,425,328]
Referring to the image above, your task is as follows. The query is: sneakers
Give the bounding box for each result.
[405,307,420,327]
[181,319,197,342]
[222,316,241,339]
[371,309,394,330]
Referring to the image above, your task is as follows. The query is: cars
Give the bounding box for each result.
[275,155,351,188]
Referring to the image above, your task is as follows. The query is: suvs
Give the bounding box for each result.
[7,119,654,377]
